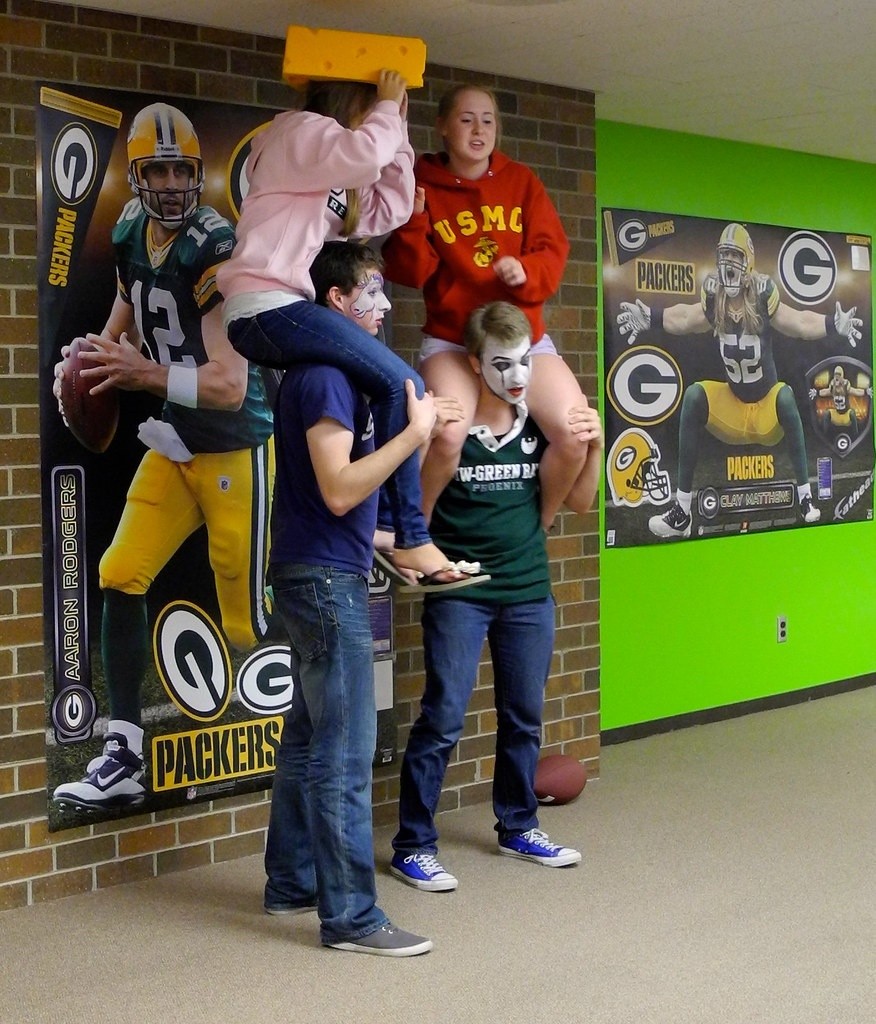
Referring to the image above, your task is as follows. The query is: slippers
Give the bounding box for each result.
[399,560,492,595]
[372,547,421,587]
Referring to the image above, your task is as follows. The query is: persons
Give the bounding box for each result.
[616,221,865,538]
[391,298,605,895]
[258,241,433,960]
[48,102,284,814]
[387,80,591,549]
[806,364,872,439]
[213,59,498,593]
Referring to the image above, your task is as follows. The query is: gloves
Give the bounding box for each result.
[864,386,874,398]
[809,388,820,400]
[825,301,863,348]
[616,299,663,346]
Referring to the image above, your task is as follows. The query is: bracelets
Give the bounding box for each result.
[166,366,198,410]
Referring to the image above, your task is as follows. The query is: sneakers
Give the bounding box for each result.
[266,906,318,915]
[327,921,433,958]
[799,494,820,522]
[499,828,582,868]
[389,851,458,891]
[52,732,146,814]
[649,500,692,539]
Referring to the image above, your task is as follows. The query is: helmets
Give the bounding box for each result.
[128,102,204,222]
[834,366,843,383]
[717,224,755,288]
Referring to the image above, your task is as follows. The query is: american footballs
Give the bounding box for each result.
[59,336,121,455]
[532,754,588,807]
